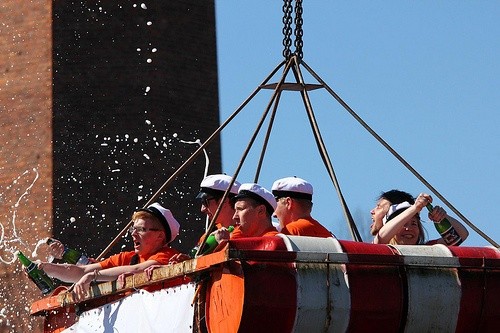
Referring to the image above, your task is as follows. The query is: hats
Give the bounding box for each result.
[136,202,180,244]
[194,173,241,198]
[271,175,314,201]
[381,200,420,225]
[231,183,277,215]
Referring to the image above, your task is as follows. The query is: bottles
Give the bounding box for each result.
[425,202,464,246]
[46,239,94,265]
[191,225,234,257]
[16,250,55,296]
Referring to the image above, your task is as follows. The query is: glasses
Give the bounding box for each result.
[127,226,160,234]
[201,197,216,207]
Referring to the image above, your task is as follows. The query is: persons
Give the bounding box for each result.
[271,176,332,238]
[370,190,415,236]
[371,192,469,246]
[23,202,180,303]
[169,183,281,262]
[118,174,242,289]
